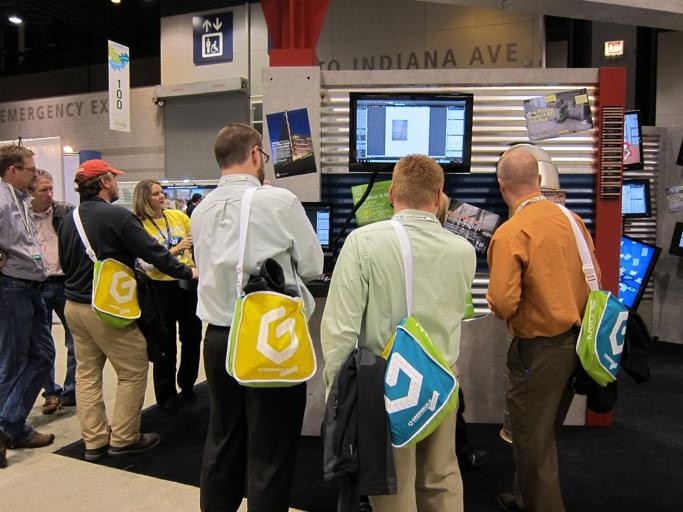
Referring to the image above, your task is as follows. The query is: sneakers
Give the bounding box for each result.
[40,391,63,416]
[82,440,109,463]
[104,431,162,458]
[7,426,56,450]
[0,429,11,469]
[56,389,75,416]
[151,381,205,417]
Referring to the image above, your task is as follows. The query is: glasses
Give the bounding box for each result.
[257,146,270,163]
[13,164,36,173]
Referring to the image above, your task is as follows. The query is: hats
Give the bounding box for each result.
[73,157,126,183]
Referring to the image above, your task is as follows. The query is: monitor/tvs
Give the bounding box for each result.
[621,179,652,217]
[622,110,645,171]
[349,92,474,175]
[617,234,661,314]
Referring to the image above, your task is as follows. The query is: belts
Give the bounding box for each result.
[0,271,45,293]
[45,274,67,283]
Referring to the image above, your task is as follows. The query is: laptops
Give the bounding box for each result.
[301,201,333,258]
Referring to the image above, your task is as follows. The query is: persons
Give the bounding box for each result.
[321,154,476,512]
[191,123,324,512]
[0,145,56,469]
[129,180,204,414]
[25,167,77,415]
[57,159,199,463]
[485,150,600,512]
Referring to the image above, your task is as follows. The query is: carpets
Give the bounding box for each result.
[51,346,683,512]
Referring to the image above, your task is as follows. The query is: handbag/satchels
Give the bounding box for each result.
[568,283,633,389]
[218,288,321,393]
[366,313,463,451]
[87,255,145,332]
